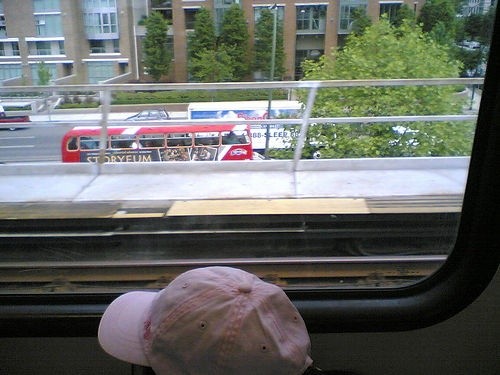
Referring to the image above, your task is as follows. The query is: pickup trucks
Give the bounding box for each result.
[0,112,30,131]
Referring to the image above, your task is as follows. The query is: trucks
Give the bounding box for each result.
[188,101,337,159]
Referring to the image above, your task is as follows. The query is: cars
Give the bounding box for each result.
[124,109,170,121]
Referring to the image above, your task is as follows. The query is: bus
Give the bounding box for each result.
[62,122,253,162]
